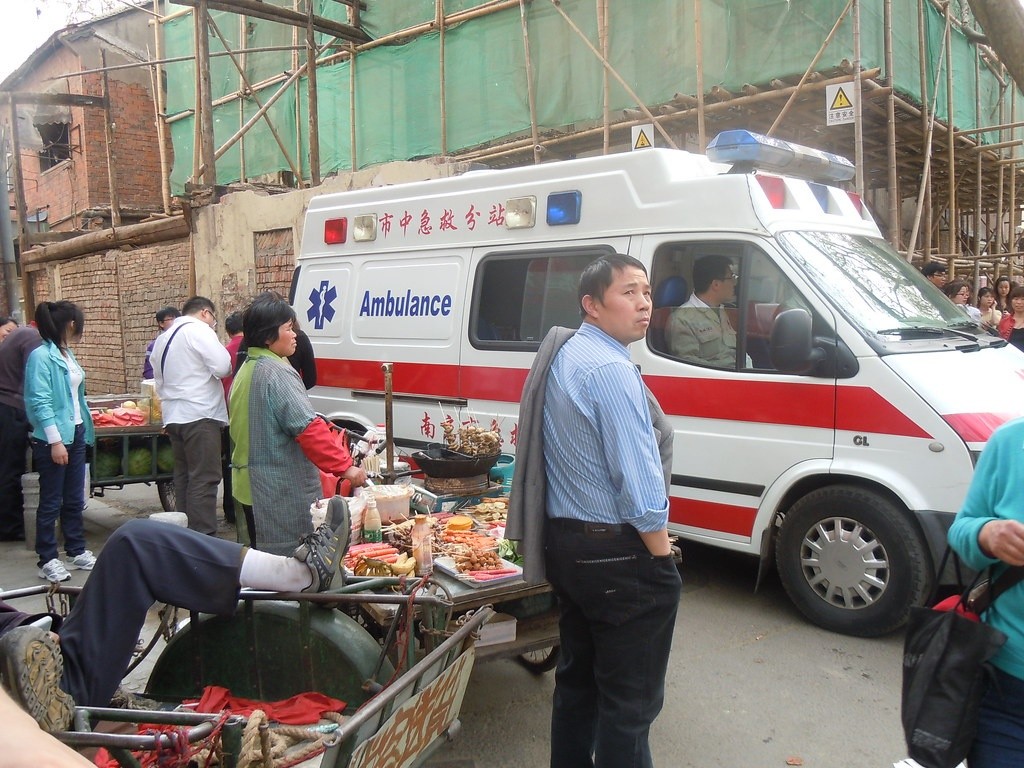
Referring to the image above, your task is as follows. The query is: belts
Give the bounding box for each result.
[555,519,638,536]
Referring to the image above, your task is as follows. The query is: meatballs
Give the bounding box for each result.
[448,545,503,572]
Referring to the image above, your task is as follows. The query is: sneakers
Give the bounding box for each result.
[38,558,71,583]
[292,495,353,608]
[65,550,97,571]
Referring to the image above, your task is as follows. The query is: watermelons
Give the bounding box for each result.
[90,449,120,478]
[121,447,153,476]
[157,442,174,473]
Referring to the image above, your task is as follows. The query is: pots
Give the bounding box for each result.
[411,443,501,478]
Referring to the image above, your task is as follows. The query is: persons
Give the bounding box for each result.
[228,298,367,557]
[977,287,1002,329]
[920,262,946,288]
[221,310,244,524]
[947,416,1024,768]
[996,285,1024,353]
[941,282,968,305]
[235,292,317,391]
[25,299,98,582]
[1011,281,1020,289]
[149,296,233,537]
[990,276,1012,314]
[664,255,753,368]
[0,495,352,768]
[142,306,181,379]
[950,278,980,319]
[542,255,681,768]
[0,320,43,540]
[0,316,19,344]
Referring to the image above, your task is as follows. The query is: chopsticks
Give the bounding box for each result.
[360,456,381,474]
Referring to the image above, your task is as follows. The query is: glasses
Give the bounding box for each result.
[162,318,175,322]
[718,274,740,280]
[935,274,945,278]
[202,309,218,328]
[957,293,968,297]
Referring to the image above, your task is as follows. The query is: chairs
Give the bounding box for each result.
[651,274,687,354]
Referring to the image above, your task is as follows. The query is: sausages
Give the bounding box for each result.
[349,543,399,564]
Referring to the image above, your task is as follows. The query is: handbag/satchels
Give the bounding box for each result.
[901,565,1008,767]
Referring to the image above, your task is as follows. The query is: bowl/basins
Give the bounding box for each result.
[365,484,414,522]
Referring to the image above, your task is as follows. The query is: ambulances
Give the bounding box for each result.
[287,128,1024,637]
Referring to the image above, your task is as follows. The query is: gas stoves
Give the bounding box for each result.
[400,477,503,515]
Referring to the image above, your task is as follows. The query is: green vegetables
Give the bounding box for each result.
[498,540,524,565]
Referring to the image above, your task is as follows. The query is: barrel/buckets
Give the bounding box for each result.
[309,497,363,548]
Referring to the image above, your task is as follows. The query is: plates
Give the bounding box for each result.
[434,556,524,588]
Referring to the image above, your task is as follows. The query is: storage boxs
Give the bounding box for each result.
[474,613,517,648]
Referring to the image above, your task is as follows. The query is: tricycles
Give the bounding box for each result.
[0,576,496,768]
[313,412,560,673]
[89,426,178,511]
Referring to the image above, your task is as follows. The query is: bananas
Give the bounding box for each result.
[353,552,416,578]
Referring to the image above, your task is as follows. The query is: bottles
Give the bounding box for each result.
[363,501,382,544]
[412,514,433,577]
